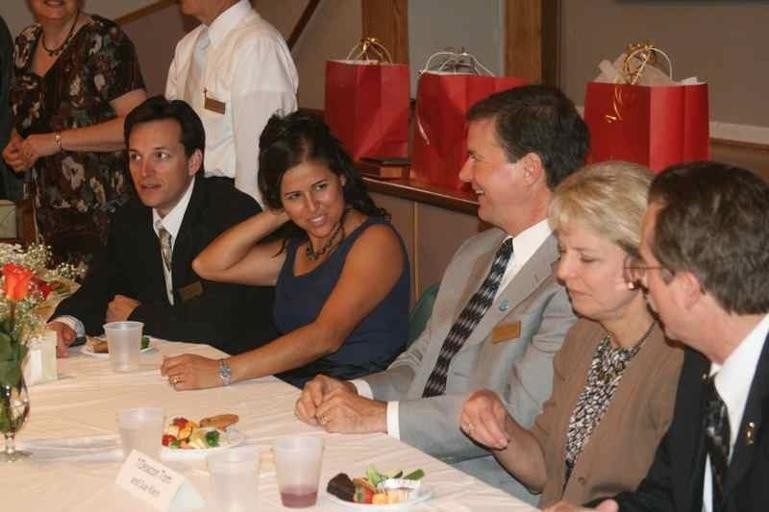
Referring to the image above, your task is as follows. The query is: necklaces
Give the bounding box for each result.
[304,215,351,266]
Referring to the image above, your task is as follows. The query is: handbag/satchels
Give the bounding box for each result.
[322,38,410,162]
[412,51,531,189]
[579,49,711,173]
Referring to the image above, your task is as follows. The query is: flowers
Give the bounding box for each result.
[0,241,89,433]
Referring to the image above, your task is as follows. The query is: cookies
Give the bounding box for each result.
[200,414,239,429]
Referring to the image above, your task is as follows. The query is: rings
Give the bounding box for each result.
[466,423,475,432]
[171,376,178,384]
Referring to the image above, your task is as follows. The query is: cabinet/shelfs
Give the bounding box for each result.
[352,172,485,318]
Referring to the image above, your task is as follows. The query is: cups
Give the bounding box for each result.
[102,320,144,372]
[116,410,165,469]
[270,436,326,509]
[22,333,59,387]
[205,448,261,510]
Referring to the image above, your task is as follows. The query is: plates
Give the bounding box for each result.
[327,480,436,509]
[163,423,245,457]
[80,335,160,356]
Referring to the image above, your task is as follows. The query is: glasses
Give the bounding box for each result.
[621,251,668,284]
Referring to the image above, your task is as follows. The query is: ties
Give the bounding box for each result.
[419,238,517,399]
[700,375,733,508]
[157,226,174,275]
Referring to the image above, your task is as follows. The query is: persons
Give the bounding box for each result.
[160,0,302,207]
[292,80,593,506]
[554,160,769,511]
[459,155,686,511]
[2,0,146,254]
[157,108,410,390]
[37,94,265,359]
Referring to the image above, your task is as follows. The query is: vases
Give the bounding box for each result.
[1,349,37,462]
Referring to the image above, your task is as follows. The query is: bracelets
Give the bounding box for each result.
[217,356,231,385]
